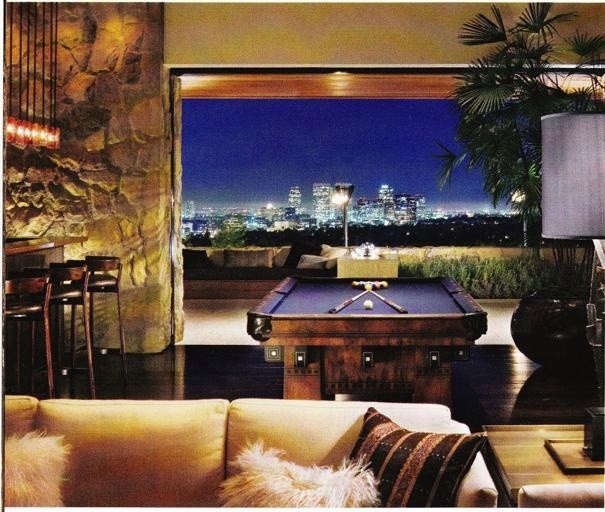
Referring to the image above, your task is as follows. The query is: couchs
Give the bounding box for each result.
[3,386,503,508]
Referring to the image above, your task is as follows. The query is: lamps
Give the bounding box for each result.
[5,3,63,152]
[538,112,605,388]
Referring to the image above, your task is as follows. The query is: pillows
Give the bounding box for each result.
[342,406,489,507]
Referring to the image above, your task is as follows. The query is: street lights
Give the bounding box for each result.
[510,187,529,248]
[334,182,354,247]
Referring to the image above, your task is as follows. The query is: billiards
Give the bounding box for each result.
[352,281,388,290]
[364,300,373,309]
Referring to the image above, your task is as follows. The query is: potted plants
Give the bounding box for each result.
[427,4,605,363]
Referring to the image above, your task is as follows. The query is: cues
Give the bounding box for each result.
[370,291,408,313]
[329,290,369,313]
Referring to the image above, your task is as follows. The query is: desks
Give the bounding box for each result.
[479,419,604,507]
[246,267,490,410]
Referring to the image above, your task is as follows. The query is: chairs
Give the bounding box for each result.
[5,230,130,399]
